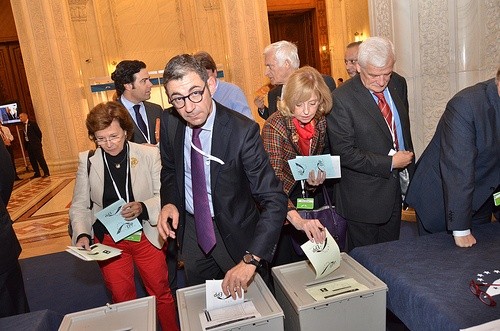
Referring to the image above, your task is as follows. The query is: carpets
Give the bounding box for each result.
[19,245,183,314]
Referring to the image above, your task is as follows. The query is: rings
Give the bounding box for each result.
[311,179,314,182]
[132,213,135,217]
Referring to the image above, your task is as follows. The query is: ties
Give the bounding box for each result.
[133,105,148,140]
[374,92,399,152]
[191,127,217,256]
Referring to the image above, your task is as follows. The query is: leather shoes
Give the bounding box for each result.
[30,173,41,178]
[43,173,50,178]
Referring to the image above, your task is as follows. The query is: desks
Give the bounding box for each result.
[0,308,52,331]
[349,220,500,331]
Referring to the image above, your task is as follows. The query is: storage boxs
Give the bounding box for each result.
[176,272,285,331]
[57,296,157,331]
[271,252,389,331]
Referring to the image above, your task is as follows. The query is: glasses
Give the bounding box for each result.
[469,279,500,307]
[168,82,207,109]
[93,129,125,145]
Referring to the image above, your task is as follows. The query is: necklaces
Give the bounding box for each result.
[108,155,126,169]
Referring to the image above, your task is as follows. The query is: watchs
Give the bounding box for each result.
[243,253,260,267]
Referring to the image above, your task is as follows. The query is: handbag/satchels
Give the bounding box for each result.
[68,203,92,239]
[291,205,347,256]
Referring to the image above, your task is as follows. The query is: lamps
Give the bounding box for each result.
[354,31,365,42]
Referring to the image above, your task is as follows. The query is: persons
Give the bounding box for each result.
[110,59,164,145]
[403,70,500,248]
[0,121,22,180]
[19,113,50,178]
[6,107,13,120]
[193,51,253,121]
[337,78,343,87]
[69,101,180,331]
[326,36,416,253]
[261,65,333,266]
[344,41,362,78]
[0,136,30,318]
[253,40,335,121]
[156,53,288,298]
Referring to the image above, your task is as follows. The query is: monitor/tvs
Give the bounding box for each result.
[0,101,24,125]
